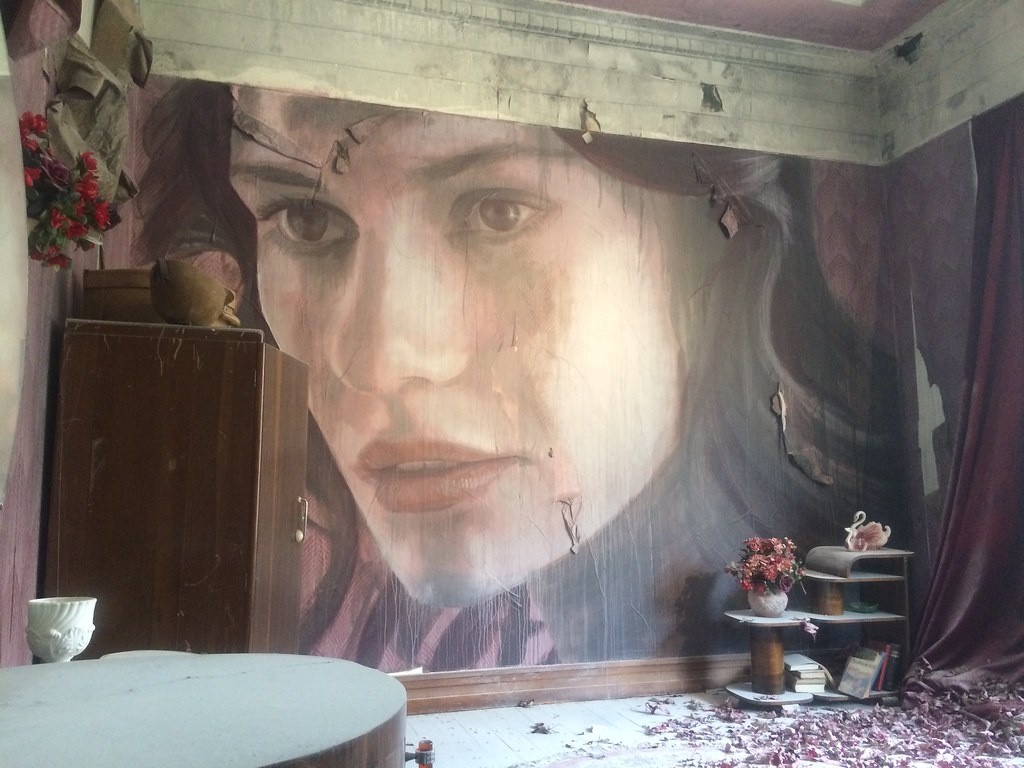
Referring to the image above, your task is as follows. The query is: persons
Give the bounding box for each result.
[139,79,905,672]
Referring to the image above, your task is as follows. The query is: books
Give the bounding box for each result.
[784,654,836,692]
[837,641,903,699]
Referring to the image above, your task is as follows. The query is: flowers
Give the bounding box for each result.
[724,537,805,595]
[20,110,113,276]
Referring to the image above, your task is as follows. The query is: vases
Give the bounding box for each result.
[748,587,789,617]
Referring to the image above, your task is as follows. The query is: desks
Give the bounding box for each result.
[723,609,813,707]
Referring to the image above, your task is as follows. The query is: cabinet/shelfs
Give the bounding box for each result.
[786,546,915,702]
[43,317,311,657]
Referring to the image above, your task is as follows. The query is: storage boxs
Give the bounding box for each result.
[84,269,159,322]
